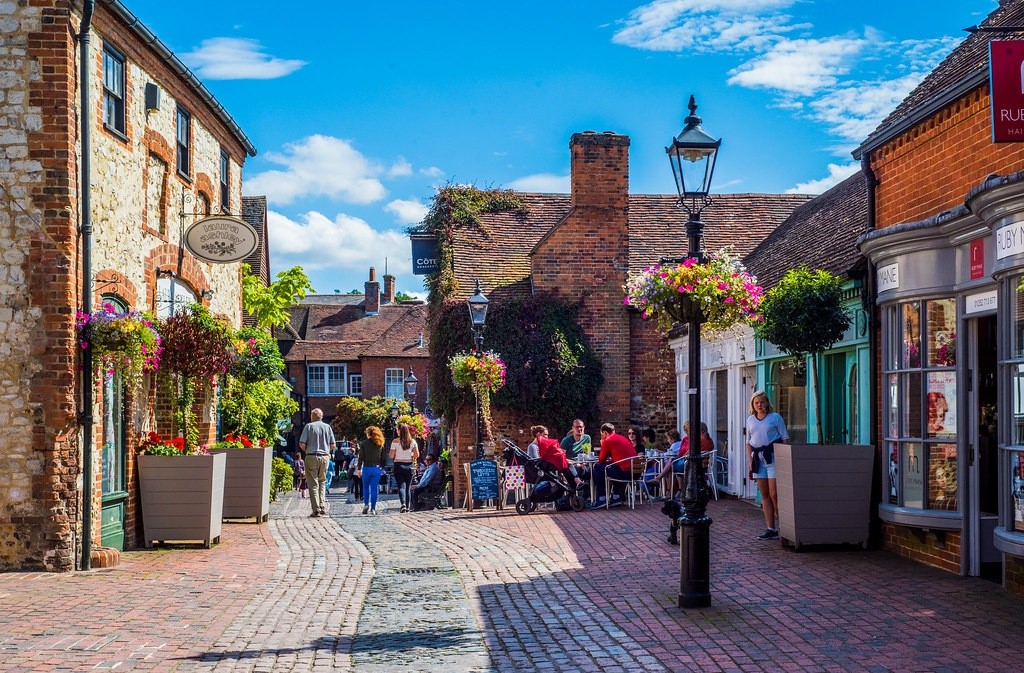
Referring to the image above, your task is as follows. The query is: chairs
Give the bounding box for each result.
[515,447,719,509]
[378,475,390,495]
[717,456,728,485]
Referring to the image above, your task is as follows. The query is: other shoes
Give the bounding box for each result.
[355,497,358,501]
[320,510,325,514]
[674,490,682,501]
[310,511,321,517]
[362,505,369,514]
[360,497,364,501]
[400,504,406,513]
[575,481,590,491]
[406,509,410,512]
[372,509,376,516]
[645,478,660,488]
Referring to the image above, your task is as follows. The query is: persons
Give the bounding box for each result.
[627,426,646,454]
[642,428,656,449]
[527,425,588,490]
[299,408,335,517]
[745,390,789,540]
[560,419,591,458]
[589,423,638,509]
[281,451,294,467]
[411,454,447,511]
[390,423,420,512]
[295,452,304,492]
[350,457,364,501]
[342,449,356,493]
[335,445,344,475]
[646,423,715,500]
[358,425,386,514]
[325,454,335,494]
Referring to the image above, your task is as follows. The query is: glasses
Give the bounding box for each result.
[425,458,433,461]
[627,432,637,435]
[573,425,585,428]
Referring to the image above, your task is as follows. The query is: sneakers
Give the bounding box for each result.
[608,494,623,507]
[756,528,779,541]
[589,497,606,510]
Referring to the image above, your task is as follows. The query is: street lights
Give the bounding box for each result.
[405,365,419,416]
[657,95,724,609]
[465,278,490,507]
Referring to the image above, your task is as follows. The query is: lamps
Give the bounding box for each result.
[202,288,214,301]
[155,266,177,281]
[146,82,160,114]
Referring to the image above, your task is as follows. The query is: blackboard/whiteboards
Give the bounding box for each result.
[388,474,398,489]
[467,460,501,499]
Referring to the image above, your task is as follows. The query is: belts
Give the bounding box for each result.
[307,452,329,457]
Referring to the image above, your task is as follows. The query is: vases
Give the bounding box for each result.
[206,446,274,524]
[99,331,128,351]
[135,451,226,549]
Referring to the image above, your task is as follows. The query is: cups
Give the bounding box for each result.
[645,448,673,458]
[577,452,595,461]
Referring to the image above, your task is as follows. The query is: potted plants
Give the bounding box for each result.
[231,325,287,384]
[581,443,593,458]
[645,443,655,457]
[753,261,875,556]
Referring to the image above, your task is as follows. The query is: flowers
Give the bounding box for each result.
[933,329,957,367]
[395,413,432,441]
[620,243,766,415]
[208,432,275,448]
[74,303,261,422]
[889,340,921,386]
[446,348,508,447]
[139,431,211,456]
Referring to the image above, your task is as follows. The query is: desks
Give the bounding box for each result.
[647,455,677,498]
[573,459,609,506]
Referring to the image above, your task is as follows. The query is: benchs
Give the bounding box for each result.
[417,475,454,511]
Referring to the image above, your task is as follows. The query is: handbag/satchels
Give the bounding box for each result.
[346,467,355,480]
[505,454,525,490]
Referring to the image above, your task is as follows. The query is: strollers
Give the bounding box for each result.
[501,438,587,515]
